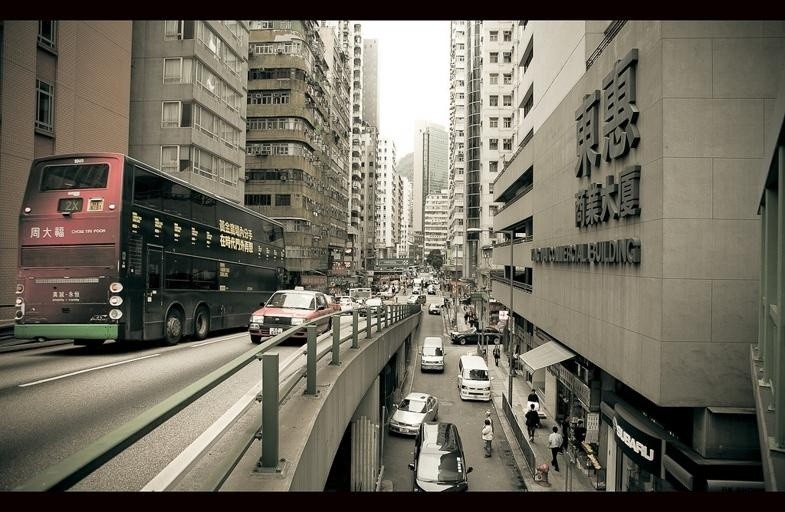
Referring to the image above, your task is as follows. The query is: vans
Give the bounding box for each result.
[457,355,495,402]
[418,336,447,372]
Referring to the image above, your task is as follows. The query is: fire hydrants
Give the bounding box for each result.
[536,463,551,486]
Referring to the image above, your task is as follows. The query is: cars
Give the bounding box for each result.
[419,263,438,287]
[372,292,393,300]
[427,287,436,295]
[428,303,440,315]
[412,287,422,293]
[372,266,418,294]
[388,392,439,437]
[427,285,434,290]
[451,326,504,345]
[358,298,388,318]
[323,293,336,306]
[246,284,333,346]
[460,296,471,304]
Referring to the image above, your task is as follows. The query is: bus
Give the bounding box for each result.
[8,152,289,347]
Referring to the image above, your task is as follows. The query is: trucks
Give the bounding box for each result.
[412,279,423,291]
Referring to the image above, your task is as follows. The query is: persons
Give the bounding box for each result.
[482,410,494,450]
[574,420,586,441]
[559,415,571,454]
[525,404,542,442]
[344,267,479,328]
[482,419,493,457]
[547,426,564,472]
[528,389,540,411]
[493,345,500,366]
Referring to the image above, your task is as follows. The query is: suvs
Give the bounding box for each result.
[407,294,427,305]
[409,418,474,492]
[335,296,358,315]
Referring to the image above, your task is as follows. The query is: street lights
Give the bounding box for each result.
[444,237,458,326]
[466,226,515,408]
